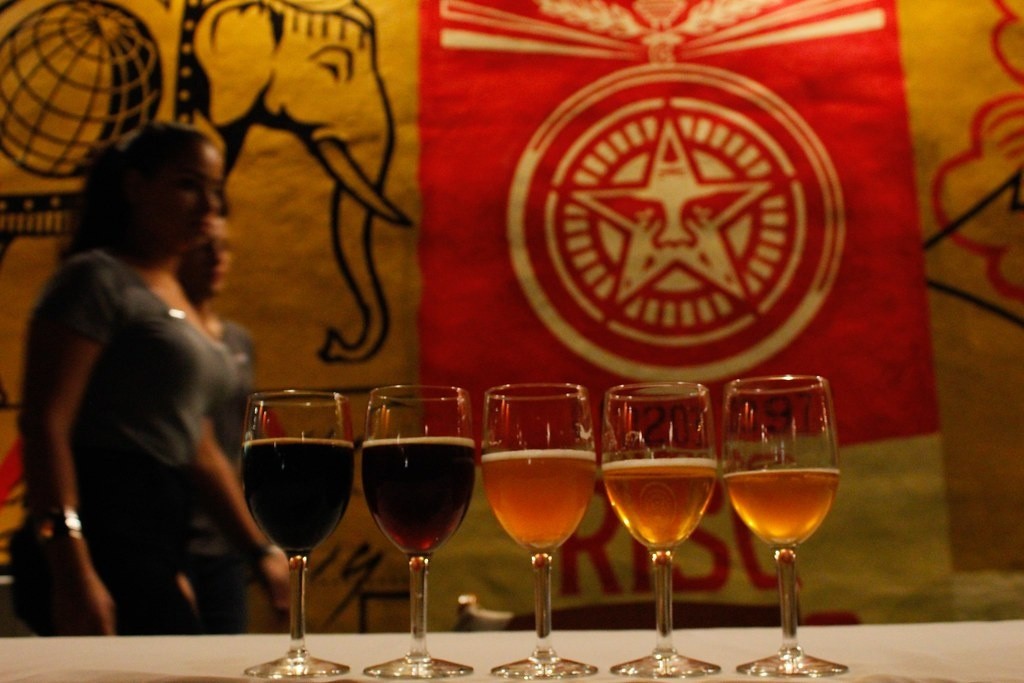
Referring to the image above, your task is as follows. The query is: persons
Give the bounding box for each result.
[7,118,291,636]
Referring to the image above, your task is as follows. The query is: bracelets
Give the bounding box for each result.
[249,541,279,565]
[26,504,80,547]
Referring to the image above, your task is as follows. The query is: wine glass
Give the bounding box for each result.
[362,386,475,680]
[239,390,356,678]
[723,377,850,679]
[602,383,722,681]
[481,384,597,680]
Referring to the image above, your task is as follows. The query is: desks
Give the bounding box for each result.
[0,618,1024,683]
